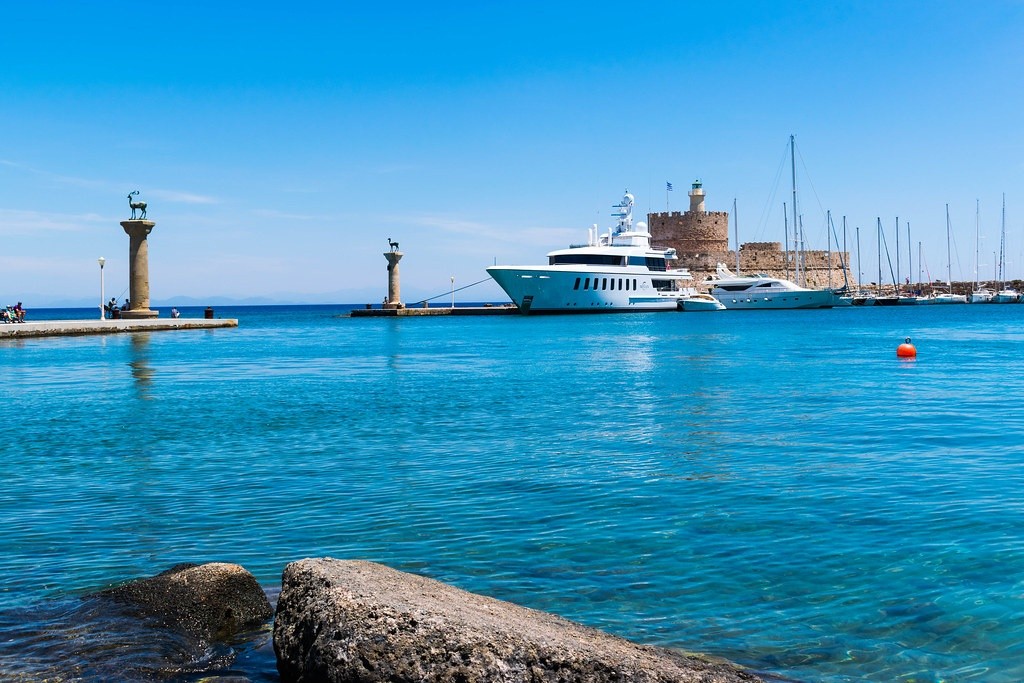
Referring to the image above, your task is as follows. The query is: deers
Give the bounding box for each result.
[126,191,147,220]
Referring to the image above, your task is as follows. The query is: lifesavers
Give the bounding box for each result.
[666,265,671,270]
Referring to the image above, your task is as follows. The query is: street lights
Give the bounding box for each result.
[450,276,456,308]
[96,257,106,322]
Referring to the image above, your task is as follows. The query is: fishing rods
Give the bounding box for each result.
[115,288,129,303]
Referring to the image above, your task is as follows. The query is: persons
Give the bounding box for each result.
[122,299,130,310]
[13,302,25,323]
[98,303,110,317]
[109,298,116,317]
[0,307,12,323]
[171,307,179,318]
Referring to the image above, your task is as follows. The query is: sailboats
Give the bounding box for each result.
[715,135,1024,308]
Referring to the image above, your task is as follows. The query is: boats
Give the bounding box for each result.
[702,261,833,309]
[486,191,700,312]
[677,292,727,311]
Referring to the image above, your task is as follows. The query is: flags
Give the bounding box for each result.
[667,182,672,192]
